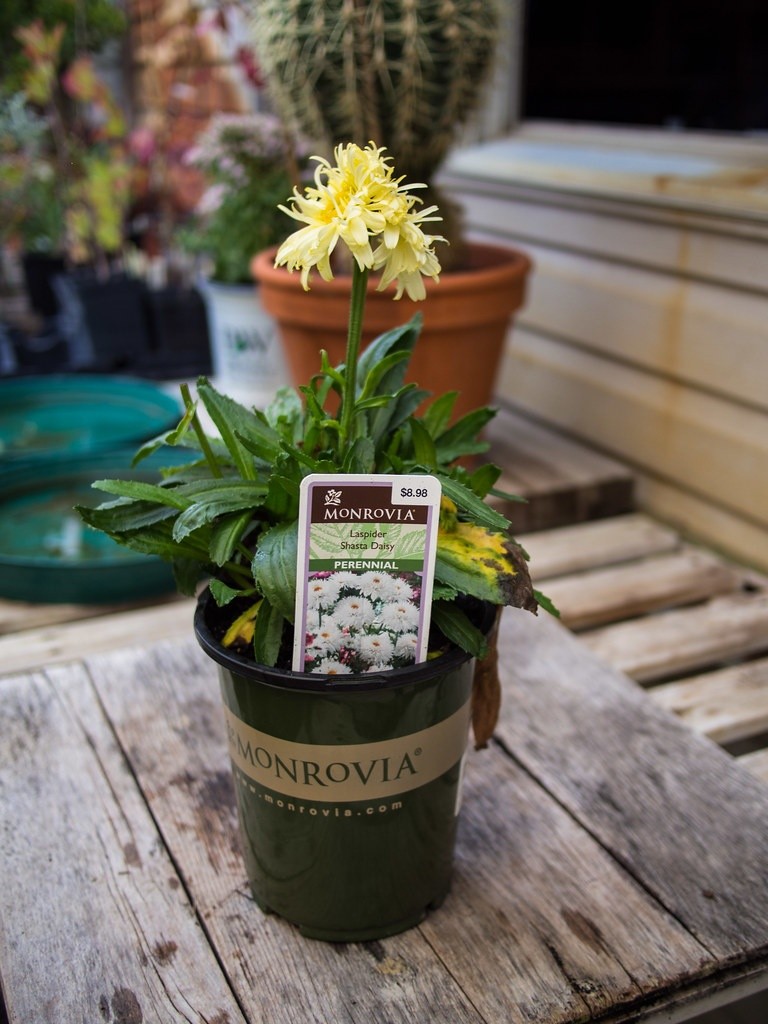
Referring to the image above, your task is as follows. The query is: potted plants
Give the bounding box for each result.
[1,1,234,382]
[243,3,536,473]
[176,131,307,394]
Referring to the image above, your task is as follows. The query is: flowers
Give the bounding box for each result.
[67,134,567,675]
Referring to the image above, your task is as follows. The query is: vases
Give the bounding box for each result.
[187,572,506,942]
